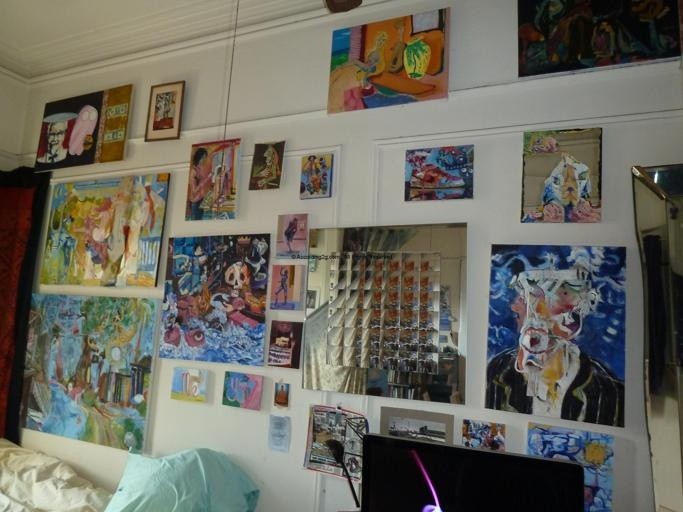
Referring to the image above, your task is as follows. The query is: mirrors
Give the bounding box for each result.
[300,223,467,410]
[626,158,682,511]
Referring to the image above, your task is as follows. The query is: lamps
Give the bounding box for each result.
[324,437,360,509]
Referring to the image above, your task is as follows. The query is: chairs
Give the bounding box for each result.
[365,366,388,396]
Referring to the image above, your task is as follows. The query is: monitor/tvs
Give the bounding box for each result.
[362,434,584,512]
